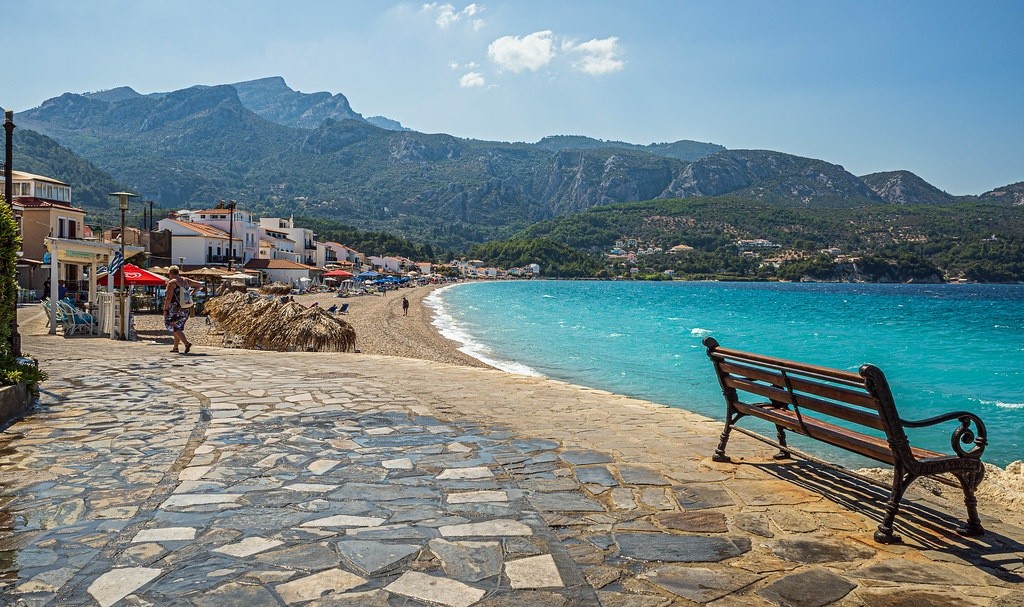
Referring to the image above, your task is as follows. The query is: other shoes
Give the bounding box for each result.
[185,342,192,353]
[170,348,178,352]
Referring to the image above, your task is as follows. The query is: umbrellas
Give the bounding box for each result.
[322,267,411,290]
[181,266,235,297]
[99,263,170,297]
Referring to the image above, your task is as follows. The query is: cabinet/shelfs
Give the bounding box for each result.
[146,296,157,311]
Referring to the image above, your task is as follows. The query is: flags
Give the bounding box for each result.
[106,247,124,275]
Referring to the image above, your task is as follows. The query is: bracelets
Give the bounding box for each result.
[164,310,169,312]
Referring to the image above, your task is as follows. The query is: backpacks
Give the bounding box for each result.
[171,279,194,309]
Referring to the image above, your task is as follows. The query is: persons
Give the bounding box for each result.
[403,297,409,317]
[70,291,86,303]
[382,286,386,297]
[163,263,202,354]
[326,303,336,312]
[39,277,51,302]
[282,295,295,304]
[58,280,67,300]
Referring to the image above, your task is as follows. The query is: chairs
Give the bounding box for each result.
[206,315,248,349]
[18,289,36,302]
[40,296,97,336]
[312,303,349,315]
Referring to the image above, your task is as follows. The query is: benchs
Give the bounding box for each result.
[702,337,989,544]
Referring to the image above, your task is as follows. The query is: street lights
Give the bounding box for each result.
[109,192,137,341]
